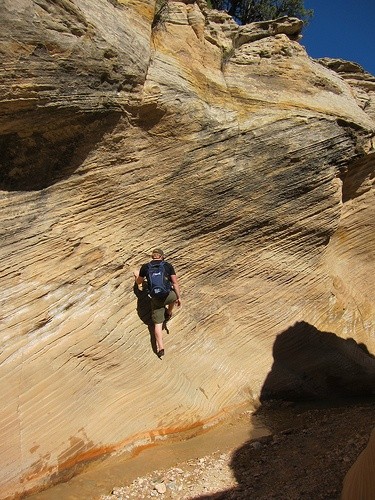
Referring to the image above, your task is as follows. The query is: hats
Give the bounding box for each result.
[152,248,164,256]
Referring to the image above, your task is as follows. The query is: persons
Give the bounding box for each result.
[133,248,182,357]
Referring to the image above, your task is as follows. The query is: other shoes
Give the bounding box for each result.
[166,310,173,318]
[158,349,165,358]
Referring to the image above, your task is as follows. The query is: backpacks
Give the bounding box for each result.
[145,260,171,299]
